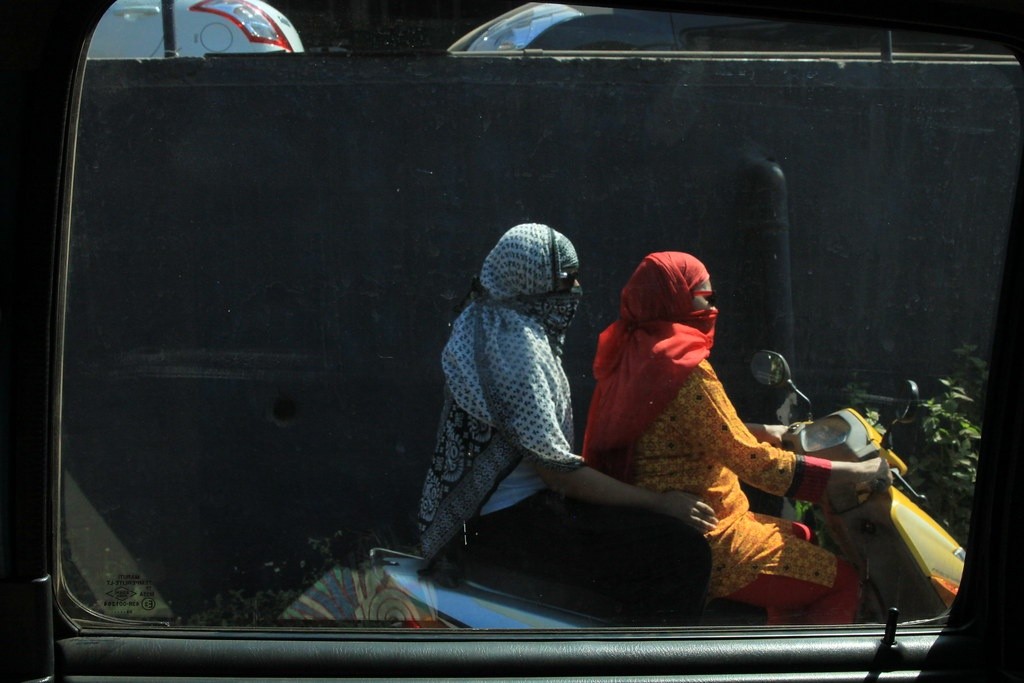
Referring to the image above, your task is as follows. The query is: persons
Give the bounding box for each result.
[581,250,892,624]
[415,222,713,625]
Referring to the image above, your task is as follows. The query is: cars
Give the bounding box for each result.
[446,2,1013,52]
[86,0,311,58]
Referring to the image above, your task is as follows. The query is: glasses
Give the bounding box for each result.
[690,288,719,306]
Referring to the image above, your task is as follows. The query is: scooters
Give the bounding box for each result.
[359,348,968,627]
